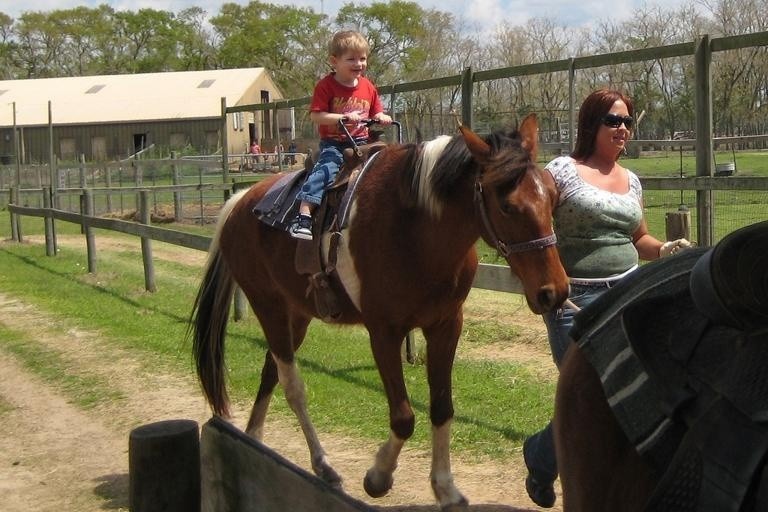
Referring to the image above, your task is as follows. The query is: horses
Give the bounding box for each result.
[175,111,574,512]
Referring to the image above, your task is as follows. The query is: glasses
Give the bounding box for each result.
[602,113,634,130]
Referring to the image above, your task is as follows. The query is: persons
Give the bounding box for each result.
[283,29,395,240]
[247,140,261,163]
[274,141,284,161]
[520,88,695,510]
[286,139,298,164]
[306,142,314,157]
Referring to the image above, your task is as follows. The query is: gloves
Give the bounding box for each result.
[659,238,691,257]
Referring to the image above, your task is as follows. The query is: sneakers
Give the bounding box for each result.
[523,438,556,507]
[286,213,314,241]
